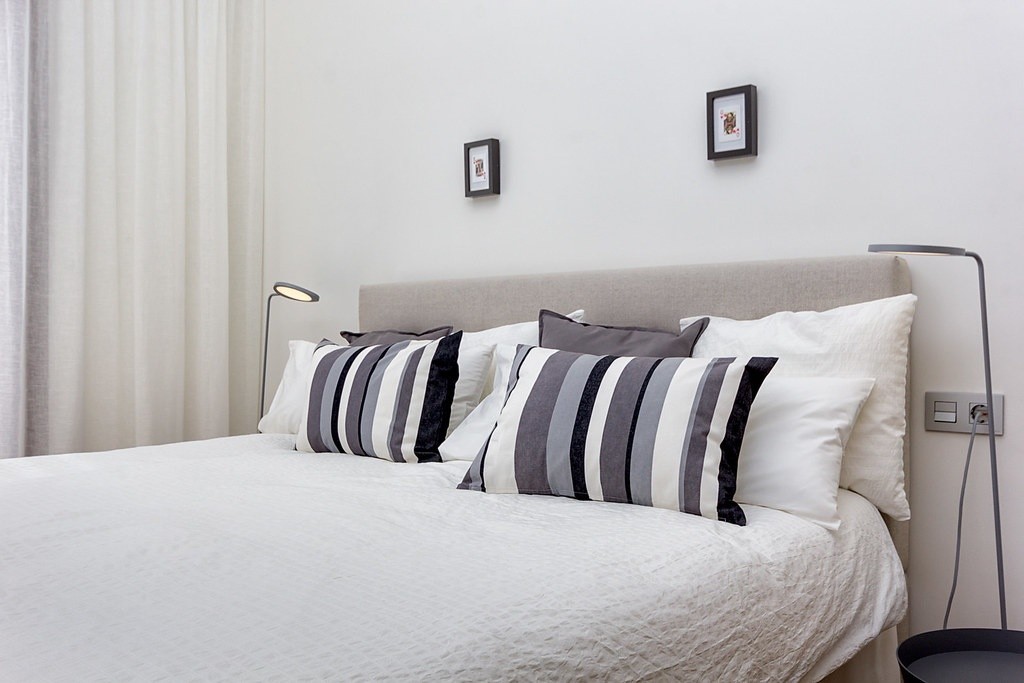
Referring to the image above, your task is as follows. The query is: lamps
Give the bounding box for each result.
[868,244,1024,683]
[261,282,320,418]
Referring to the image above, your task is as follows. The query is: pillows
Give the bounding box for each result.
[538,308,709,358]
[456,344,780,528]
[436,309,584,463]
[292,330,465,465]
[733,376,876,531]
[339,326,453,346]
[257,341,318,434]
[679,293,918,523]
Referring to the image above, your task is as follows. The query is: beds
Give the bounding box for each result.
[0,254,915,683]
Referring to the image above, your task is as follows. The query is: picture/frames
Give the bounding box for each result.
[707,85,758,158]
[464,138,500,197]
